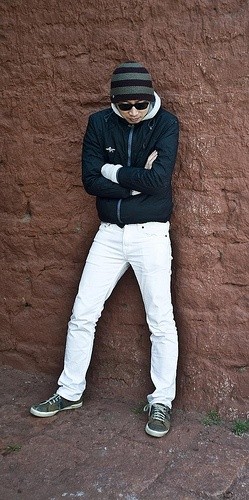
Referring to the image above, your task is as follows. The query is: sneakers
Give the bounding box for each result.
[144,403,171,437]
[30,393,84,417]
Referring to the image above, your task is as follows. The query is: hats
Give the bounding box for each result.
[109,60,155,103]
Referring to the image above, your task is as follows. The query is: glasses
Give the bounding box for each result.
[116,100,151,111]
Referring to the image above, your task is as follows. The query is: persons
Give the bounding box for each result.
[30,61,186,438]
